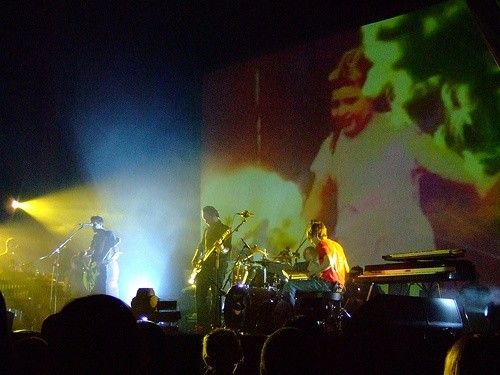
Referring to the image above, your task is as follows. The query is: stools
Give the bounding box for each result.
[314,291,343,332]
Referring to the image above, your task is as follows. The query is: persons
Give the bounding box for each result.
[300,47,499,268]
[303,245,317,262]
[78,215,120,298]
[191,205,232,334]
[0,237,22,270]
[278,222,350,310]
[0,294,500,375]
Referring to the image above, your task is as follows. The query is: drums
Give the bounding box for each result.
[223,285,288,333]
[266,263,291,287]
[233,263,263,286]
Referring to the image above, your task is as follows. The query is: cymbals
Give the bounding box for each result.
[270,255,300,259]
[241,238,251,250]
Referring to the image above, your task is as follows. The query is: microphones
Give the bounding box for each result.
[241,238,251,250]
[236,211,255,217]
[79,223,94,226]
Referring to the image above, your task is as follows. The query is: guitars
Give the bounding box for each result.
[83,237,121,291]
[190,228,231,284]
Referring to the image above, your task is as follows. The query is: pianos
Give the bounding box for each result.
[356,260,481,284]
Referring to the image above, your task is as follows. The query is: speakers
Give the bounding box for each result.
[352,293,463,330]
